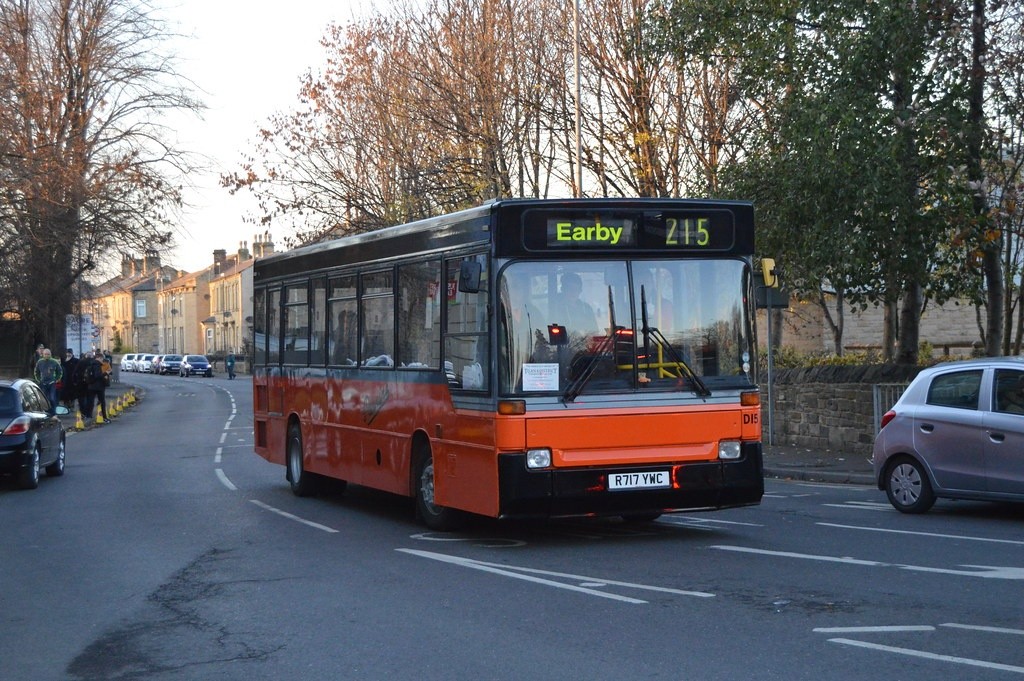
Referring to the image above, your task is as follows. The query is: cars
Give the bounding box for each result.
[180,356,212,378]
[0,379,67,489]
[872,356,1023,513]
[121,354,183,375]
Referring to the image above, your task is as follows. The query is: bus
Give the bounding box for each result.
[249,198,790,533]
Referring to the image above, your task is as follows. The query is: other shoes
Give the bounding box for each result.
[233,375,236,379]
[227,378,232,380]
[103,418,110,422]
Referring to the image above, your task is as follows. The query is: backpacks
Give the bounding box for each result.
[83,362,99,383]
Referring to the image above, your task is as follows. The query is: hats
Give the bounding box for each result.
[38,344,44,349]
[65,349,73,354]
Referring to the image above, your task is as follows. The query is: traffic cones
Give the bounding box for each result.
[94,401,106,425]
[75,409,85,432]
[105,387,136,418]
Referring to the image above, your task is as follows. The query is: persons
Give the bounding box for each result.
[555,273,599,343]
[477,272,541,389]
[333,308,358,365]
[226,350,237,380]
[31,344,112,422]
[633,269,675,331]
